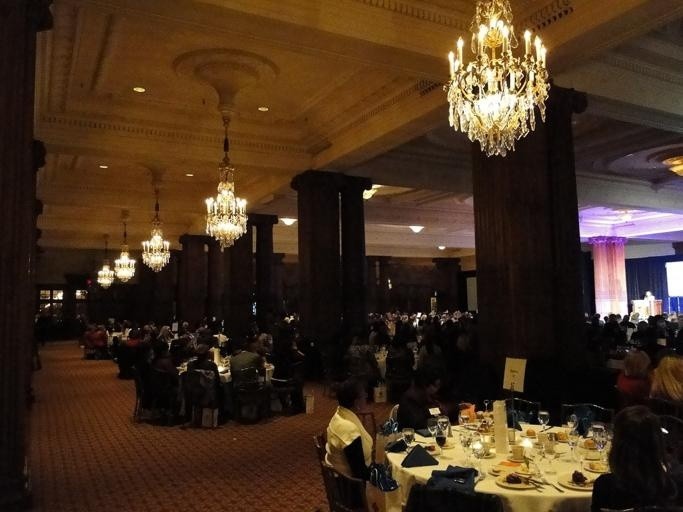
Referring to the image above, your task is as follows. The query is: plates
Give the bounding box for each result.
[557,476,593,490]
[495,475,538,488]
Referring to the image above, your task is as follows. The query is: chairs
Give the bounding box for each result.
[79,335,300,426]
[313,398,683,512]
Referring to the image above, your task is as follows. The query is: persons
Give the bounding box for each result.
[33,312,79,342]
[324,378,398,510]
[590,313,682,510]
[397,372,441,432]
[343,316,477,380]
[644,291,655,300]
[79,312,316,422]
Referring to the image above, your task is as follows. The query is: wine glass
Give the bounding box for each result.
[398,399,609,473]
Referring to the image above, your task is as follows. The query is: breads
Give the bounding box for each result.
[589,462,601,469]
[584,439,595,447]
[506,474,521,483]
[526,428,535,435]
[573,470,585,483]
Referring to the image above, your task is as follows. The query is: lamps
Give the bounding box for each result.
[173,49,279,250]
[114,210,136,282]
[97,235,114,290]
[443,0,550,157]
[647,147,683,175]
[138,158,178,274]
